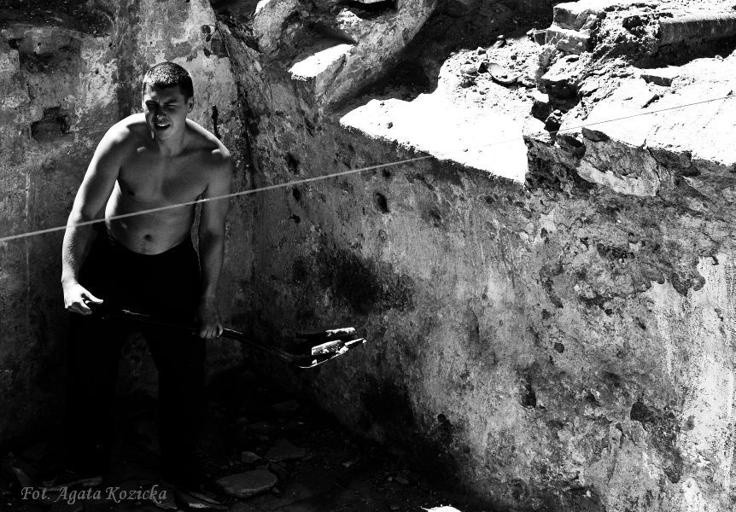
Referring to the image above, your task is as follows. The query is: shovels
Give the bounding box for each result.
[76,300,367,369]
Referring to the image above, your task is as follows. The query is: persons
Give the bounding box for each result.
[61,62,232,512]
